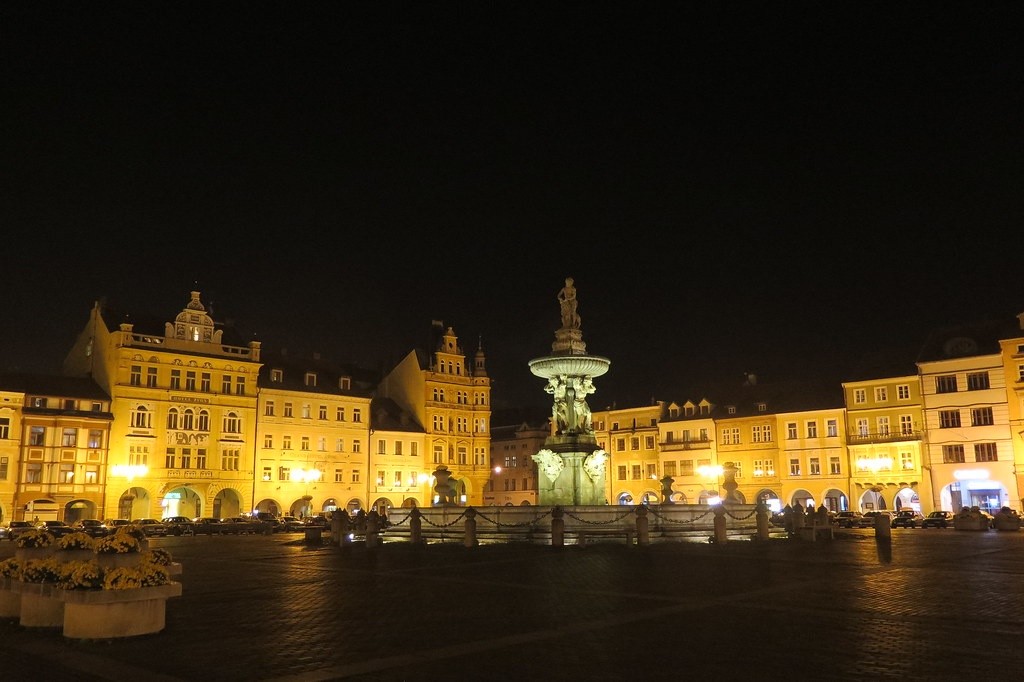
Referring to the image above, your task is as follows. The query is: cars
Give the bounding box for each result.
[300,516,328,530]
[277,516,306,532]
[100,520,141,537]
[133,519,168,536]
[832,511,862,528]
[220,518,258,534]
[161,516,200,536]
[7,520,36,541]
[892,510,925,529]
[953,511,995,528]
[194,518,234,535]
[34,521,75,539]
[72,519,109,539]
[859,510,894,528]
[922,511,955,529]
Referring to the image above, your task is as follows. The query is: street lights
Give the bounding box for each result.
[291,468,321,515]
[858,460,893,511]
[111,465,148,519]
[695,465,724,492]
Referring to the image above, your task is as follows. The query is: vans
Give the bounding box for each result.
[312,511,353,530]
[240,512,280,532]
